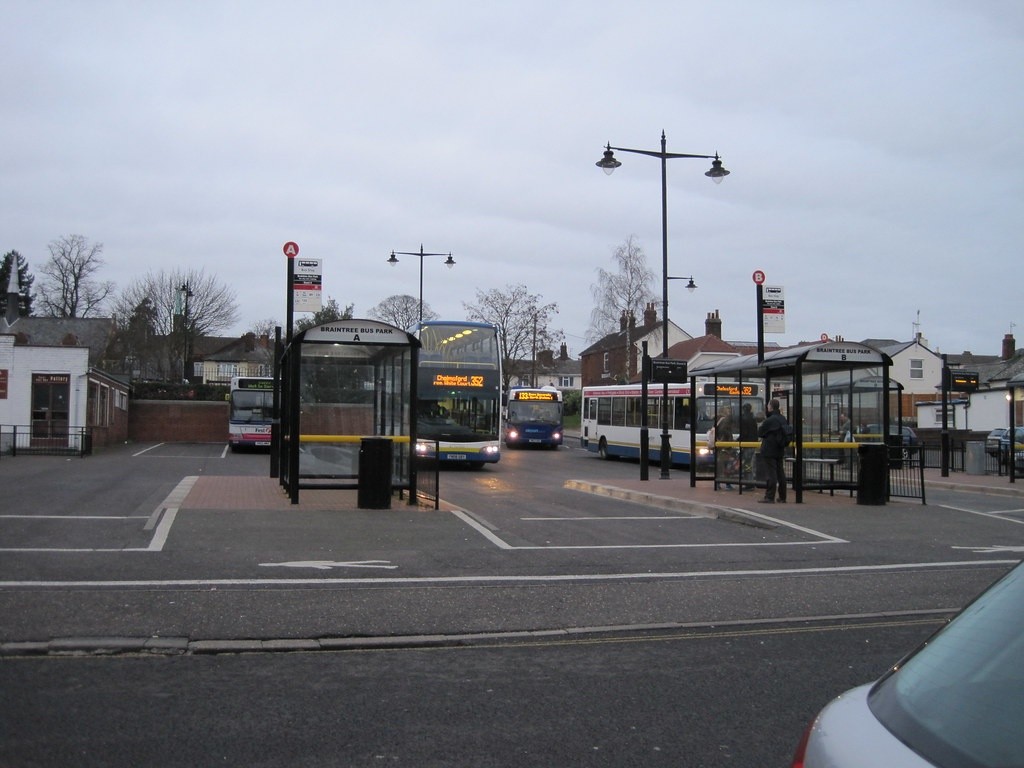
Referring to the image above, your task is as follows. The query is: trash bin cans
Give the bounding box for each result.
[857,442,889,506]
[357,437,394,508]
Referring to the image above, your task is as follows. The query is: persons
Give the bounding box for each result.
[758,400,787,504]
[736,404,758,491]
[837,413,868,470]
[713,406,733,490]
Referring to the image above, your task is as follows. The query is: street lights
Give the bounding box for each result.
[387,244,457,322]
[180,280,194,378]
[595,128,731,479]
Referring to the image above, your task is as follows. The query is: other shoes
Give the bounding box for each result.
[841,466,851,471]
[742,487,755,491]
[725,485,734,491]
[758,497,775,503]
[717,484,721,490]
[776,498,786,503]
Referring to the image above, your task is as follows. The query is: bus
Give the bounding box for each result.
[378,320,507,472]
[581,382,772,472]
[500,385,564,450]
[224,376,281,453]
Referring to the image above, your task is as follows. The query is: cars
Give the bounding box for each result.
[986,427,1024,475]
[866,424,920,459]
[790,557,1022,767]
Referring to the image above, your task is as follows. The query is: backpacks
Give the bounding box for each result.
[774,415,793,449]
[706,416,726,450]
